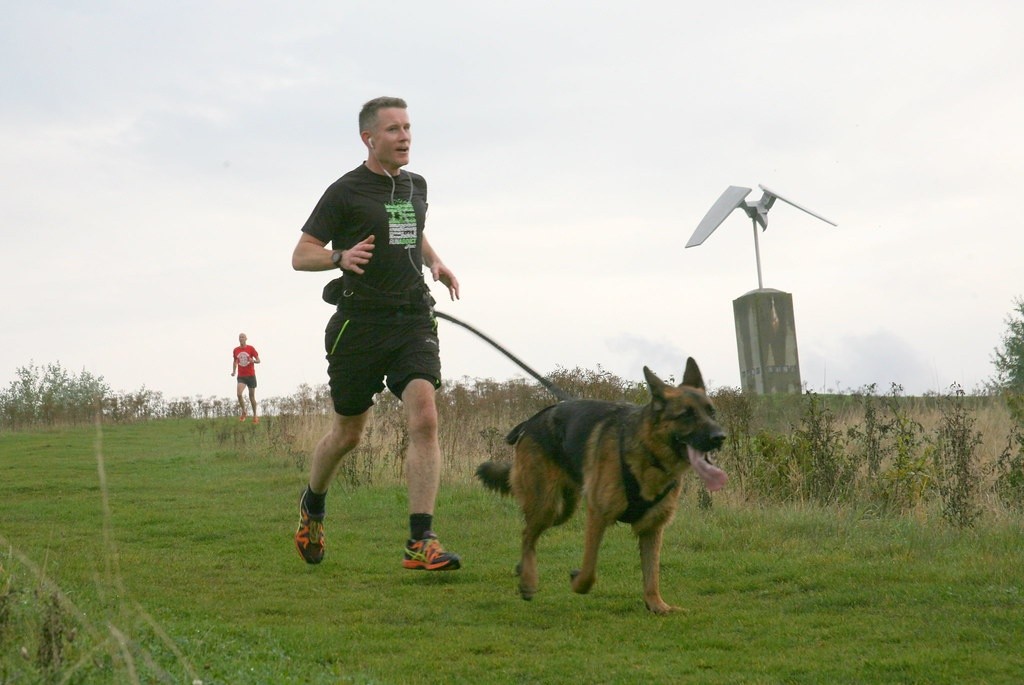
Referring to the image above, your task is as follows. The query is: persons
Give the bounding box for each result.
[291,95,462,571]
[230,333,260,425]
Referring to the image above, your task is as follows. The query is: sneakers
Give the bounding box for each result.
[239,412,247,422]
[252,418,260,425]
[402,530,461,571]
[295,489,327,565]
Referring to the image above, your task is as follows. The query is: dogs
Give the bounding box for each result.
[472,355,729,617]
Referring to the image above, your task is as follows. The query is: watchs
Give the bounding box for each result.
[331,249,346,271]
